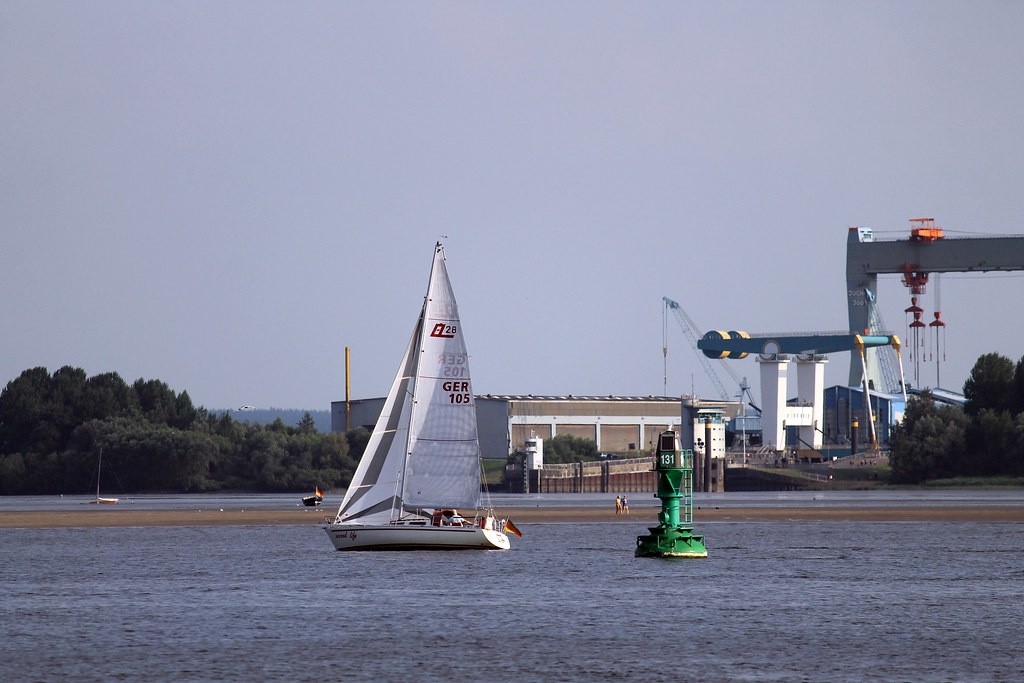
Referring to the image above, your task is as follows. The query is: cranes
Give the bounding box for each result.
[661,297,762,418]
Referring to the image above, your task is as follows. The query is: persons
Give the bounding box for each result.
[849,458,878,468]
[615,494,629,515]
[445,509,473,527]
[431,508,442,527]
[745,449,796,465]
[819,457,824,463]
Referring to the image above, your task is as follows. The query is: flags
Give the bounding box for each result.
[502,517,523,538]
[316,486,323,499]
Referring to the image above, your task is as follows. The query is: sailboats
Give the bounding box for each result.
[80,446,134,504]
[325,233,524,551]
[302,486,324,507]
